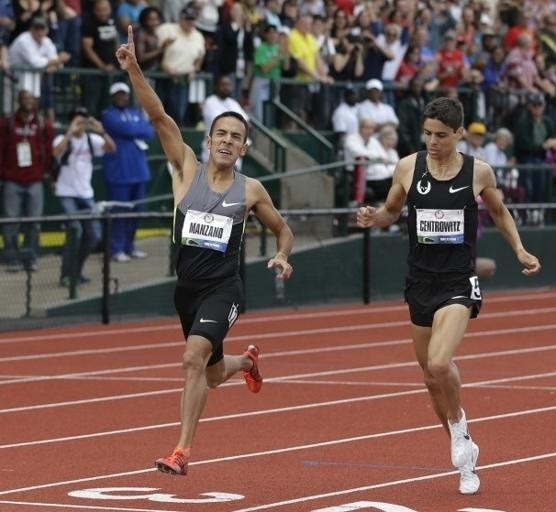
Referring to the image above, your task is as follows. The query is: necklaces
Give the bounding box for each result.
[419,155,462,180]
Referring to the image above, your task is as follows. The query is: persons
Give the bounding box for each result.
[0,0,556,234]
[0,88,55,272]
[102,82,152,264]
[354,97,545,497]
[112,23,296,478]
[52,106,115,287]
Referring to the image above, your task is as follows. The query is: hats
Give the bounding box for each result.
[365,79,384,91]
[457,35,468,45]
[469,121,487,136]
[31,15,48,28]
[179,7,196,18]
[108,81,131,98]
[529,92,546,104]
[191,5,220,33]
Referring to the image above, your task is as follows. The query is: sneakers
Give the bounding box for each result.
[241,343,264,394]
[60,274,92,288]
[457,441,481,495]
[4,260,20,271]
[24,263,38,271]
[112,251,132,264]
[154,444,192,475]
[447,408,469,468]
[131,250,148,259]
